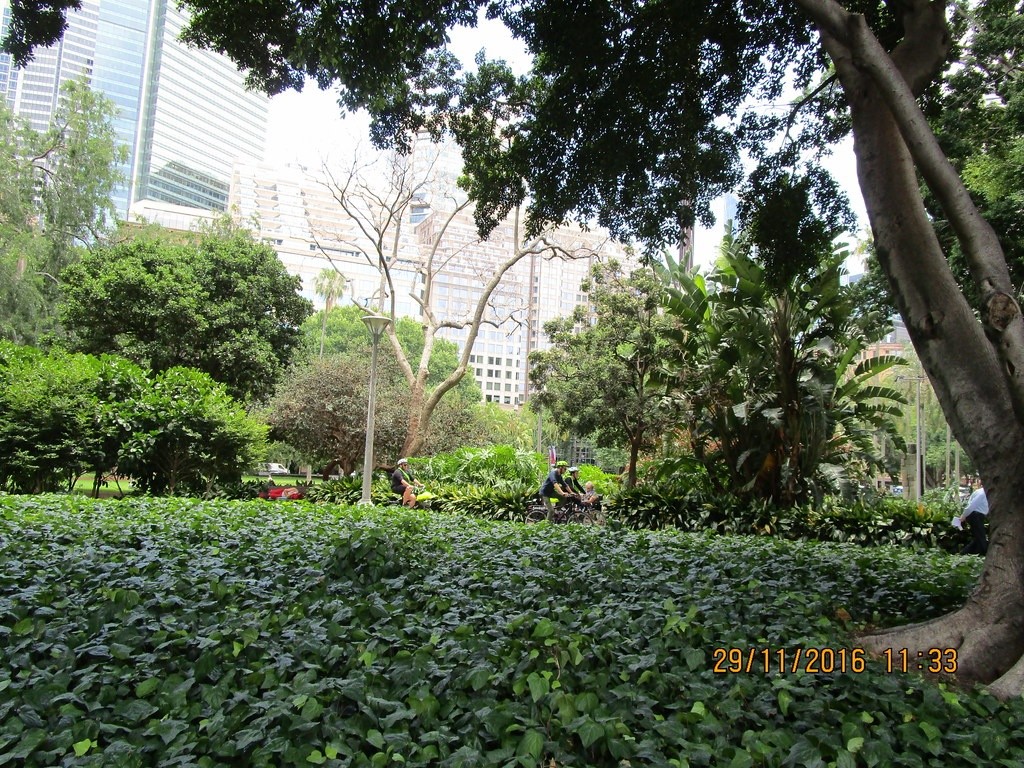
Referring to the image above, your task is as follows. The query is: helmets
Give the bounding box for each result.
[569,468,579,472]
[398,459,407,465]
[557,461,568,466]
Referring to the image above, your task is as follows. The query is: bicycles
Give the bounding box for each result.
[524,493,606,528]
[389,486,432,510]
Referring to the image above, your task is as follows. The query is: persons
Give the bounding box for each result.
[338,465,344,480]
[391,458,424,509]
[538,461,576,522]
[582,481,599,510]
[565,467,586,509]
[958,488,989,556]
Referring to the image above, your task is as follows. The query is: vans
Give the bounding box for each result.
[258,463,290,475]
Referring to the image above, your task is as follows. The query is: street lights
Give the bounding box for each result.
[895,376,923,505]
[357,316,392,503]
